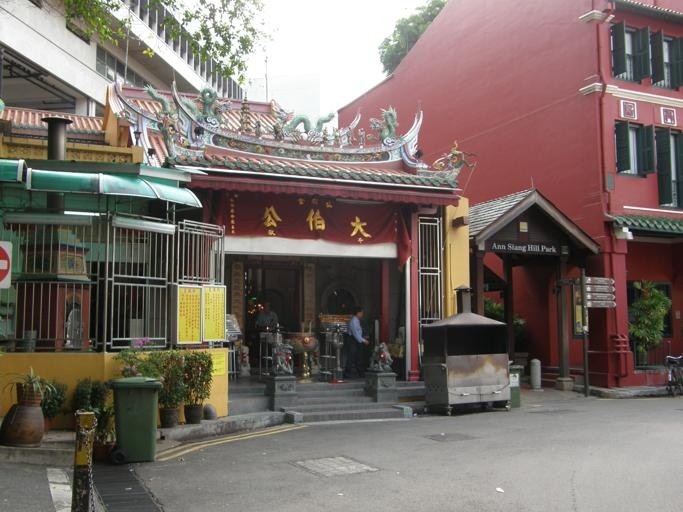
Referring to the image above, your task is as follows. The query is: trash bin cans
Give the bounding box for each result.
[509,365,525,408]
[108,376,164,465]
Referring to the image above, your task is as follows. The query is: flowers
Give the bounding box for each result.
[111,337,156,377]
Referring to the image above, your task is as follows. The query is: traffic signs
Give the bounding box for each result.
[585,284,617,294]
[586,301,617,310]
[584,276,616,285]
[585,293,617,302]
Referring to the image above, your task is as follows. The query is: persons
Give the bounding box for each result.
[343,305,370,379]
[253,299,279,364]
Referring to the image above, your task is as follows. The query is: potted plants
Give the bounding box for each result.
[182,348,217,424]
[84,402,115,461]
[40,384,68,432]
[0,366,57,407]
[144,348,190,427]
[514,317,528,352]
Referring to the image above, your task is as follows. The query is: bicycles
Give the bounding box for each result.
[664,355,683,397]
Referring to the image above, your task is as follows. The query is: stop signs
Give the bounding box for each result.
[0,241,14,290]
